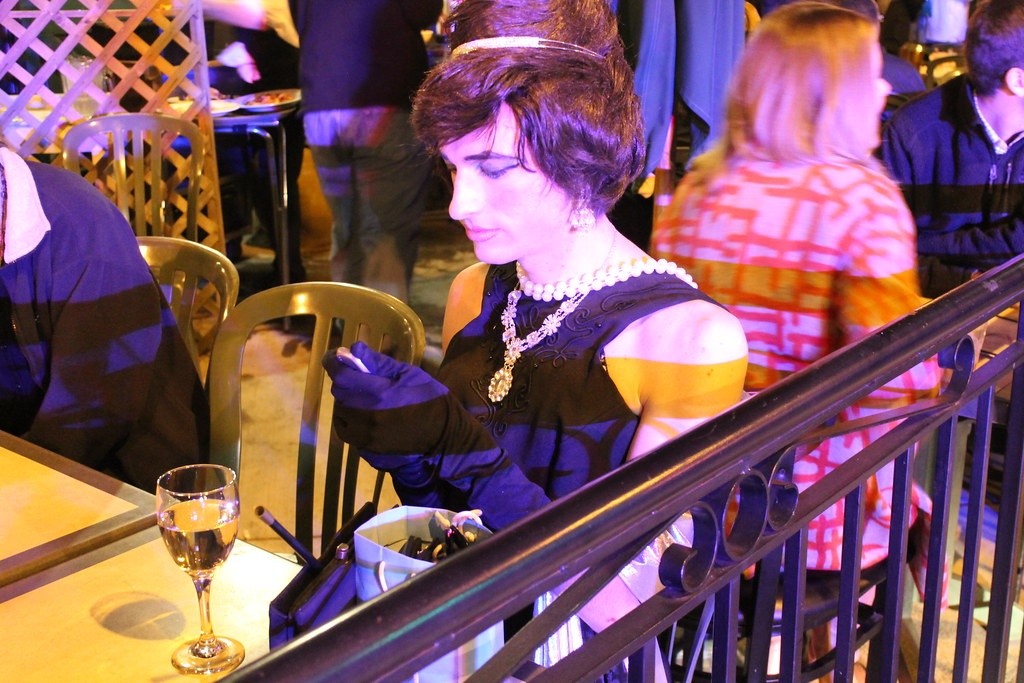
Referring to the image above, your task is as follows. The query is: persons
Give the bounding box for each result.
[842,0,927,94]
[880,0,971,57]
[871,0,1024,269]
[321,0,750,683]
[0,144,206,499]
[288,0,444,334]
[205,0,306,284]
[650,0,949,683]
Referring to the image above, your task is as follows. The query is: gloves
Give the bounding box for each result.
[322,341,551,533]
[358,450,460,509]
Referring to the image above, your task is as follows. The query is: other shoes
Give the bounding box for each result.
[226,235,244,264]
[270,257,307,281]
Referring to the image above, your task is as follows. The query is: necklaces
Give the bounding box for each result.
[488,223,618,402]
[516,259,699,305]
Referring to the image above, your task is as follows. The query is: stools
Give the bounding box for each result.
[660,541,915,683]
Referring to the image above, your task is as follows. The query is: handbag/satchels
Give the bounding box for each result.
[352,504,504,683]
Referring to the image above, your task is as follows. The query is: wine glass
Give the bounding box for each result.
[155,464,246,675]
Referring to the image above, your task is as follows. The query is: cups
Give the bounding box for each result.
[59,57,114,121]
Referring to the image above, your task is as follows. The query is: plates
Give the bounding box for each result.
[169,100,240,117]
[234,89,302,112]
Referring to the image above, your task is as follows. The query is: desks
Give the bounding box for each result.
[1,429,159,587]
[0,95,297,331]
[0,524,304,683]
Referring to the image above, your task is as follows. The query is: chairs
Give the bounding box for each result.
[62,113,205,242]
[201,282,426,566]
[136,235,240,388]
